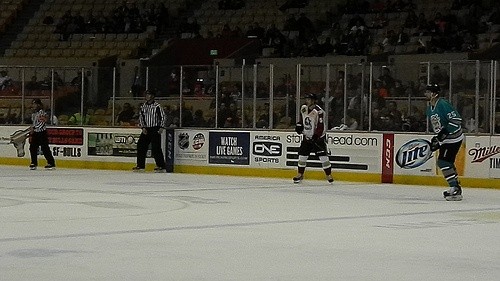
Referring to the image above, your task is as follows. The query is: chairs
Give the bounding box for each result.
[0,0,500,133]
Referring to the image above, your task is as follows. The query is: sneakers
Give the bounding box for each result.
[132,166,145,173]
[443,184,463,201]
[293,175,303,183]
[45,164,55,170]
[327,174,334,184]
[154,168,166,173]
[29,164,36,169]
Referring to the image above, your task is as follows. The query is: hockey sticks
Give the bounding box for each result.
[302,131,331,155]
[2,130,30,140]
[422,151,432,162]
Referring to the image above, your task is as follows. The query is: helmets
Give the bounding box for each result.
[304,93,317,100]
[145,90,155,96]
[32,98,41,104]
[426,82,442,92]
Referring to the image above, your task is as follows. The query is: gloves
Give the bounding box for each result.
[310,138,319,147]
[438,128,449,141]
[295,122,304,135]
[430,136,440,151]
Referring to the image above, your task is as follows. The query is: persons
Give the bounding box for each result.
[176,85,270,128]
[132,89,166,170]
[27,98,56,168]
[116,101,173,126]
[292,92,334,182]
[245,0,500,62]
[276,66,500,131]
[66,106,89,125]
[0,71,12,90]
[24,71,88,97]
[56,1,169,33]
[191,23,243,40]
[424,83,464,196]
